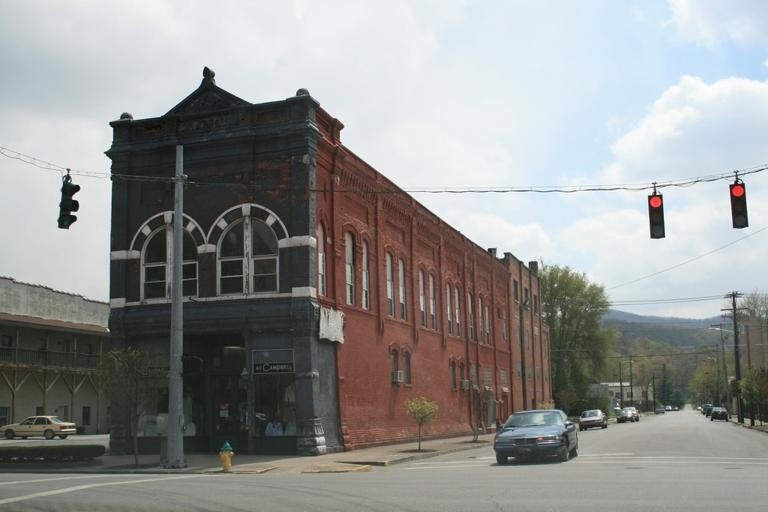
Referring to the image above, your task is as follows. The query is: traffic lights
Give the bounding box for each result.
[731,184,749,228]
[55,181,79,230]
[649,194,665,238]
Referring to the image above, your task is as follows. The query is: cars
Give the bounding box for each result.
[615,407,640,423]
[578,409,607,431]
[666,404,680,411]
[493,409,578,464]
[654,405,665,414]
[697,404,729,422]
[0,416,79,440]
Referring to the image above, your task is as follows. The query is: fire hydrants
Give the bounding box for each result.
[219,440,234,472]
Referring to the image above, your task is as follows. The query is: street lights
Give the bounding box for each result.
[706,325,756,427]
[619,360,634,409]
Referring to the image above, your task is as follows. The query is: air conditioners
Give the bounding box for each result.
[392,370,404,384]
[462,378,471,391]
